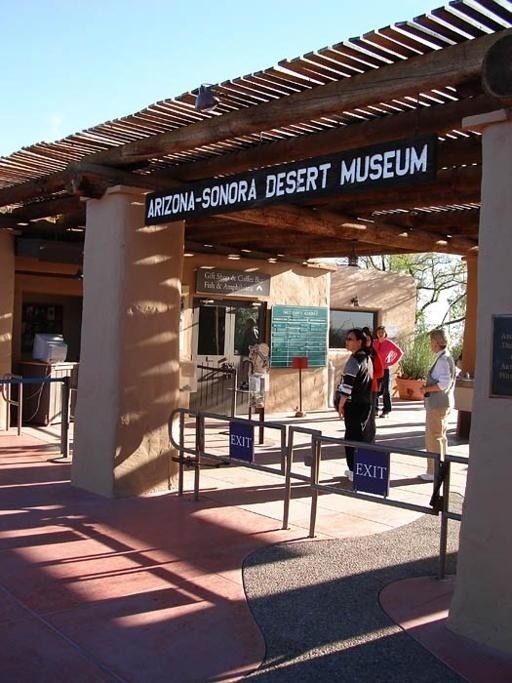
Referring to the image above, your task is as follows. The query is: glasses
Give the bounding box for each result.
[346,337,352,341]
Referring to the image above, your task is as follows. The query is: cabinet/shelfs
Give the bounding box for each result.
[20,361,76,424]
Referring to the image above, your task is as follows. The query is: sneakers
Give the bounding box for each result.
[379,412,389,418]
[420,474,434,481]
[344,470,353,482]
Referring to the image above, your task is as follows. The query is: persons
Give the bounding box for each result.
[372,326,403,418]
[419,329,456,481]
[239,318,259,355]
[248,338,269,413]
[361,327,384,416]
[336,329,375,482]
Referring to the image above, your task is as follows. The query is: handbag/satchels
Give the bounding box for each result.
[334,384,354,413]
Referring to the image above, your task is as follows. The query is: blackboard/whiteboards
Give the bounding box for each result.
[196,268,272,297]
[269,304,330,368]
[488,314,512,398]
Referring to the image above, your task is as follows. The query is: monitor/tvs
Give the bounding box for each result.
[33,333,68,364]
